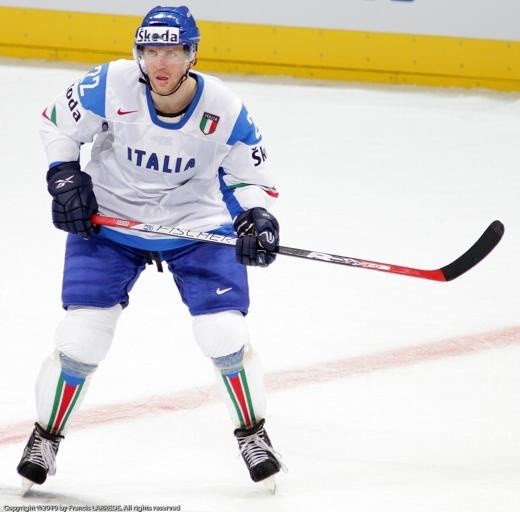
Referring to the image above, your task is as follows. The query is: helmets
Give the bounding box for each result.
[133,5,200,66]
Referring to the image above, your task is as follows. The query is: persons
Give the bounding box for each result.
[13,2,285,483]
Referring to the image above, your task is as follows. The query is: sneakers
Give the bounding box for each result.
[234,419,280,481]
[17,423,65,484]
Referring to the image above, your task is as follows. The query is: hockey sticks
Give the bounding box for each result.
[90,214,504,283]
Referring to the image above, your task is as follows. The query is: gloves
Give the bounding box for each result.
[231,206,279,267]
[46,160,101,242]
